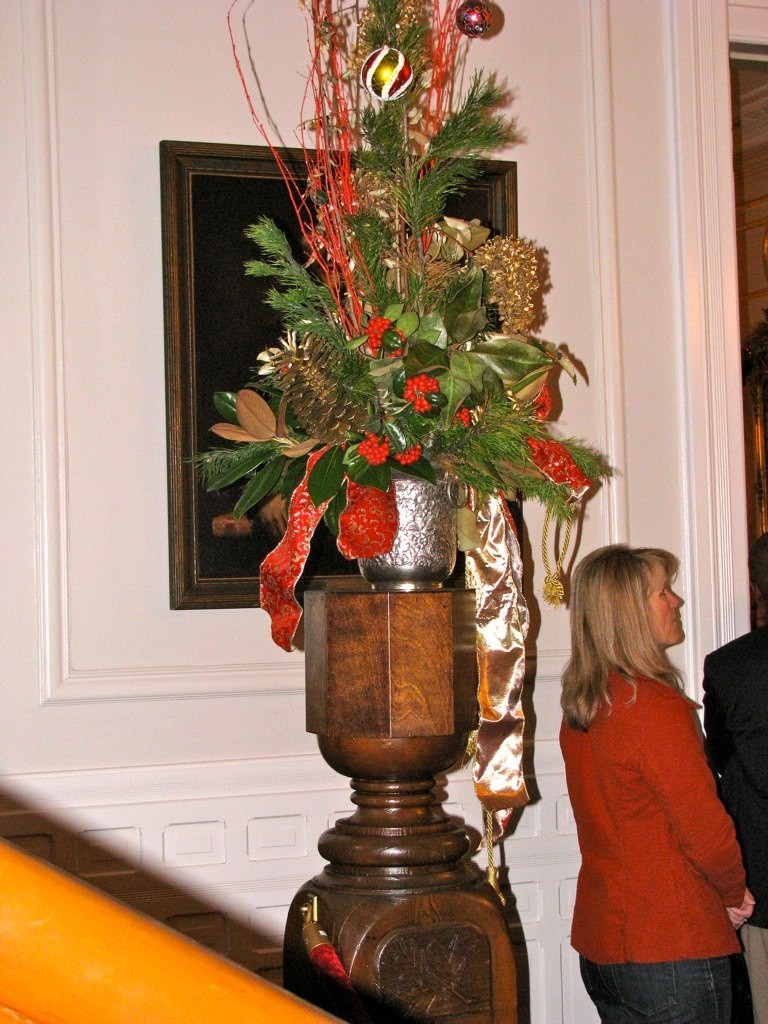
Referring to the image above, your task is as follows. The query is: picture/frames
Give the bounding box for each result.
[157,139,520,612]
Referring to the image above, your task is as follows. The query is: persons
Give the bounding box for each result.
[703,533,768,1024]
[559,546,755,1024]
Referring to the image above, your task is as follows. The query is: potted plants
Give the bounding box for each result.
[189,0,614,593]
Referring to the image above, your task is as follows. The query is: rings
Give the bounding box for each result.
[743,917,747,922]
